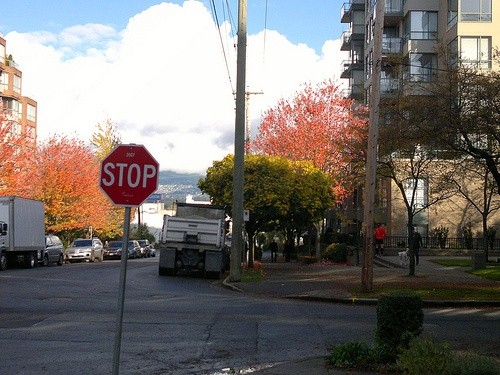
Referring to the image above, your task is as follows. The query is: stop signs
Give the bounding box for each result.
[98,144,160,207]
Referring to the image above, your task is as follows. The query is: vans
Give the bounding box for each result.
[41,236,65,268]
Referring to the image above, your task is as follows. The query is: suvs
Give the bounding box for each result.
[65,238,102,263]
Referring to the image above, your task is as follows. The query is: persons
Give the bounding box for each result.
[412,227,423,265]
[284,240,292,262]
[269,239,278,262]
[374,222,387,257]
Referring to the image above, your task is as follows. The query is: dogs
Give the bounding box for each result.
[398,248,410,266]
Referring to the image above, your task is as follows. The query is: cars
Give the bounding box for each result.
[103,242,123,260]
[126,241,140,258]
[148,244,157,256]
[138,239,149,258]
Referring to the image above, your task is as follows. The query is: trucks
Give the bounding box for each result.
[158,200,230,280]
[0,195,46,271]
